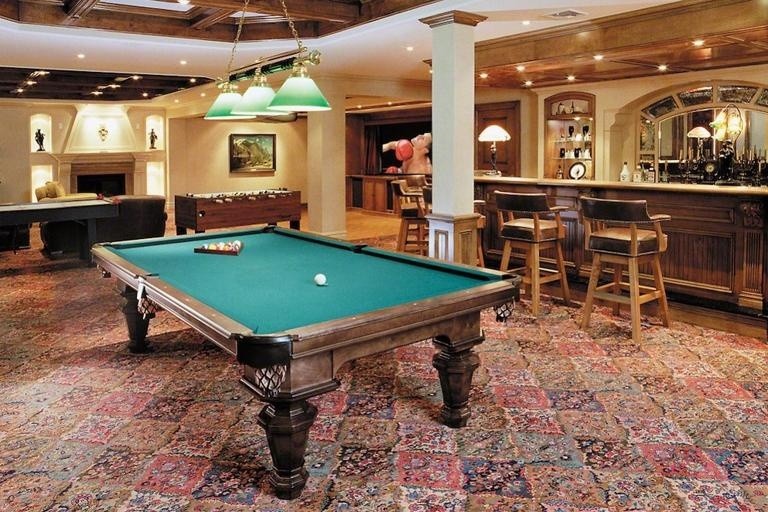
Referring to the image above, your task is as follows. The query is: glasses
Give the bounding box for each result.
[706,101,750,186]
[203,1,335,123]
[477,124,513,178]
[686,124,711,161]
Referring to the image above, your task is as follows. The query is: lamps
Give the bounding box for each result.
[389,174,488,269]
[574,192,676,344]
[492,188,571,326]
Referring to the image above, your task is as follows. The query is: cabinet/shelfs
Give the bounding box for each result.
[555,163,563,178]
[554,101,576,115]
[619,159,669,182]
[557,142,590,159]
[558,123,589,141]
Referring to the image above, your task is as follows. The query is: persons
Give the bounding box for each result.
[382,132,432,176]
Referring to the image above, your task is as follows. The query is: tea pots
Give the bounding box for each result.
[656,106,768,162]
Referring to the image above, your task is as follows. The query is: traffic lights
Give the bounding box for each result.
[44,181,58,198]
[55,183,65,197]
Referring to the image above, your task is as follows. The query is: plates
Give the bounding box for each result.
[361,177,388,213]
[543,90,597,179]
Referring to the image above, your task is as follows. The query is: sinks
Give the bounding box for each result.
[34,180,97,205]
[35,193,169,253]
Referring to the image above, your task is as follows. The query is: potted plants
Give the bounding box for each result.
[229,132,279,174]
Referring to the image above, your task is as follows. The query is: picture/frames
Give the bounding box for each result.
[88,224,527,500]
